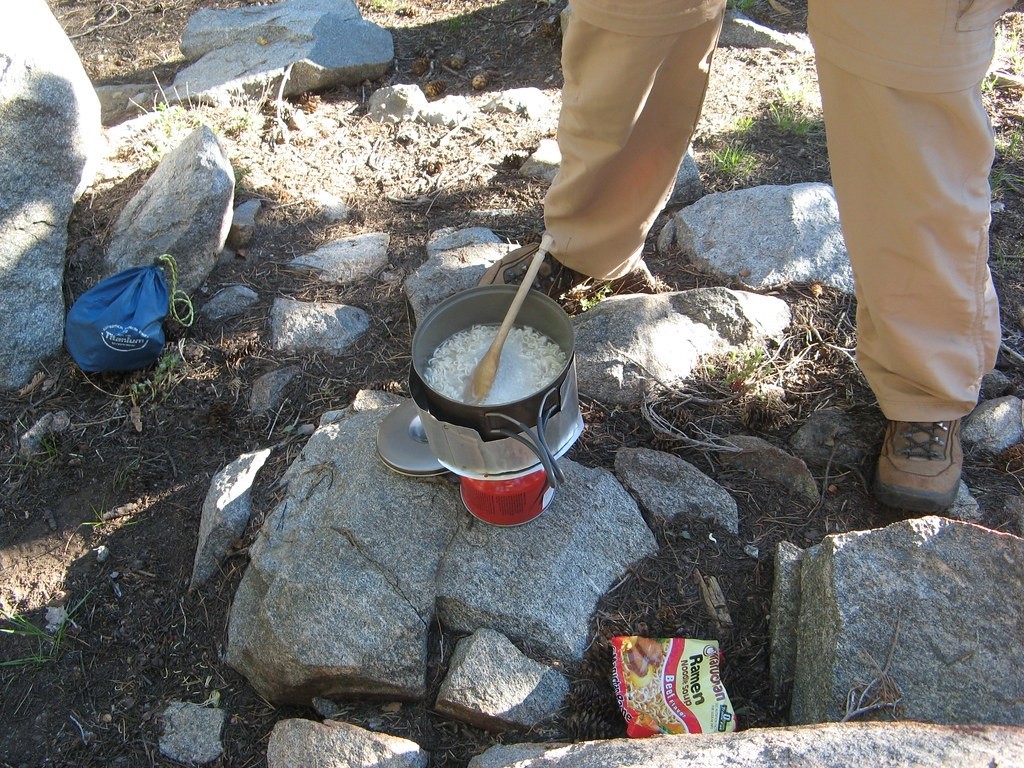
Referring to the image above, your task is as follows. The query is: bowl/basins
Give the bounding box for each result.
[460,471,555,528]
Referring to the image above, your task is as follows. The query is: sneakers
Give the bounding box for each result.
[874,419,964,513]
[475,241,592,300]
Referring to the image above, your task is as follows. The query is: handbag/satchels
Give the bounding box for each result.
[65,254,194,372]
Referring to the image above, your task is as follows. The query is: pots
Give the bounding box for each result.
[409,285,586,481]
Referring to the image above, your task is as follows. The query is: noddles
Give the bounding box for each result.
[425,322,567,406]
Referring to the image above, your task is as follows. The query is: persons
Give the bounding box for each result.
[479,0,1017,515]
